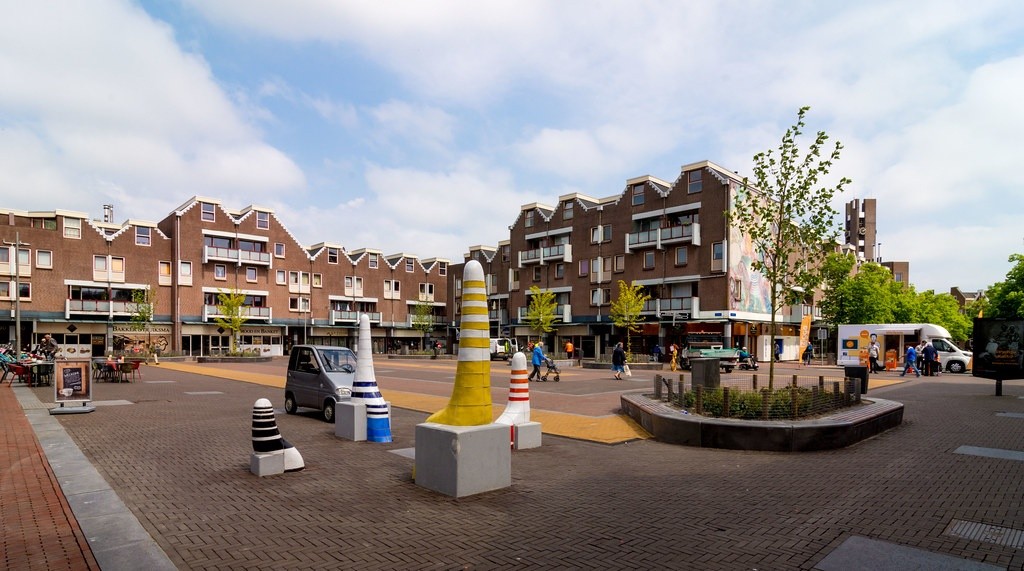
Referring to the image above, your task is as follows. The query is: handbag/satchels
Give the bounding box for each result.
[623,364,632,376]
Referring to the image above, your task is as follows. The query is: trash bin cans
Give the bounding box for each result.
[846,365,870,394]
[827,353,835,365]
[688,357,721,390]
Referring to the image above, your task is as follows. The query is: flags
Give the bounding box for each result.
[455,328,460,340]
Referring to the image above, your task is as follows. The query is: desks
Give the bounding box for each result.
[20,362,54,387]
[104,363,126,383]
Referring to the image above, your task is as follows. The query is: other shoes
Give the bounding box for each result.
[528,377,532,381]
[536,379,543,382]
[614,374,622,380]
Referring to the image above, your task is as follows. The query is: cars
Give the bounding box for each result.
[284,345,357,424]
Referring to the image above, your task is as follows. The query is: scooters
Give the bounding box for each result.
[739,354,760,371]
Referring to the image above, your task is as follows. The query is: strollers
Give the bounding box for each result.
[541,357,561,382]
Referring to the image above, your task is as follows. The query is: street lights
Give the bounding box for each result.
[298,295,311,344]
[3,231,31,362]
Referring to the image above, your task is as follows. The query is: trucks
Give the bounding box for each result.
[837,323,973,374]
[679,331,740,373]
[490,338,519,361]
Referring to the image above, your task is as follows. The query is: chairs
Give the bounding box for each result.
[94,360,143,383]
[0,357,54,388]
[299,355,315,372]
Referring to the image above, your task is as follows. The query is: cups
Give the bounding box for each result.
[59,389,73,397]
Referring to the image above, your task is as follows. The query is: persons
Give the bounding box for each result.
[145,341,160,365]
[565,340,574,359]
[40,334,58,360]
[527,342,548,382]
[432,339,442,359]
[740,347,759,367]
[804,342,812,365]
[653,344,660,361]
[869,342,879,373]
[669,344,677,372]
[921,342,938,376]
[774,340,780,363]
[915,339,927,371]
[899,344,921,377]
[612,342,627,379]
